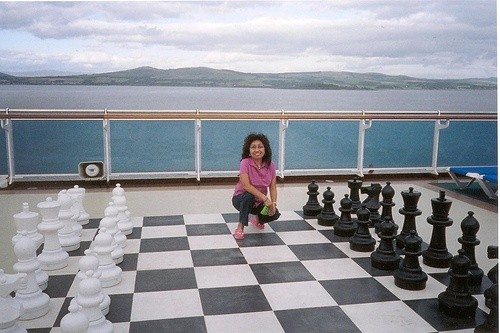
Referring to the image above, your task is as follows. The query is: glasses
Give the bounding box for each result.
[250,146,265,150]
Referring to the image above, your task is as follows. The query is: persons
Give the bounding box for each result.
[231,134,281,239]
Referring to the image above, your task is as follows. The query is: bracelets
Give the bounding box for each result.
[271,201,277,206]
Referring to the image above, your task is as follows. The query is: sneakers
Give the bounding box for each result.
[234,229,244,239]
[251,217,263,230]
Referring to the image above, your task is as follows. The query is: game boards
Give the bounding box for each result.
[4,211,493,333]
